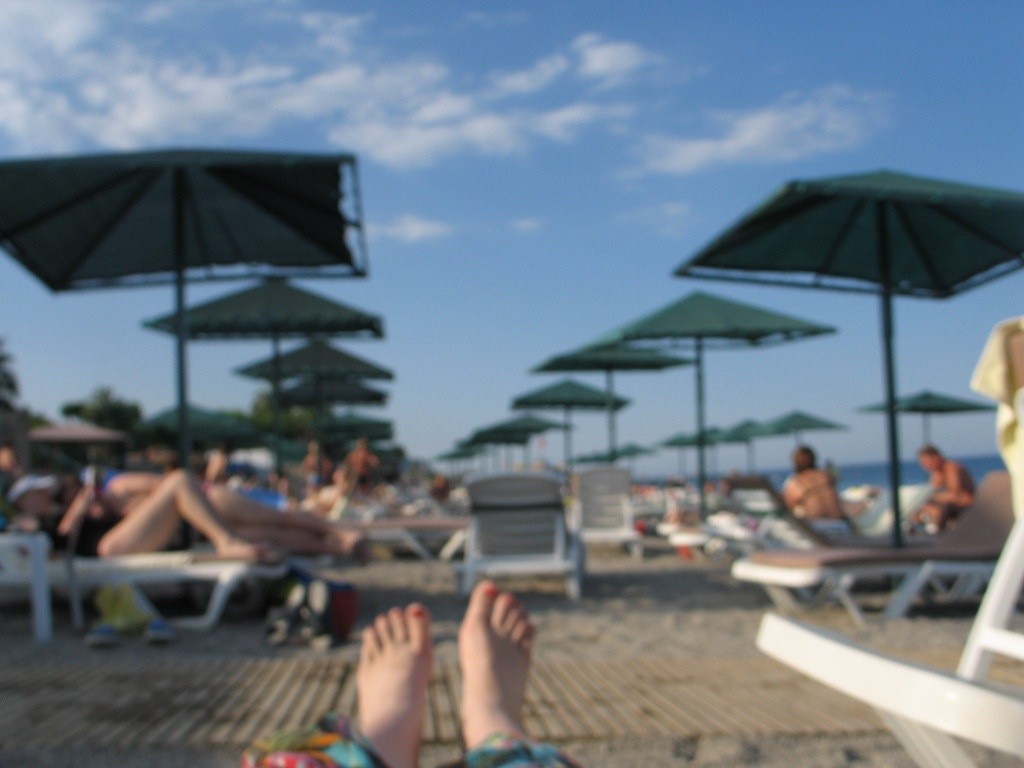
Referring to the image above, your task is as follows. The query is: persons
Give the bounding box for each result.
[242,583,579,768]
[0,436,452,650]
[917,445,972,531]
[784,447,860,530]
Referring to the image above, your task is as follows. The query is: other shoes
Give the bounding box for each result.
[143,617,174,641]
[86,624,116,646]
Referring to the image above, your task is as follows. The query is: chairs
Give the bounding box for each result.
[0,460,1024,767]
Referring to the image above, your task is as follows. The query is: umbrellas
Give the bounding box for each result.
[0,146,366,464]
[139,274,849,497]
[863,389,998,444]
[677,170,1024,542]
